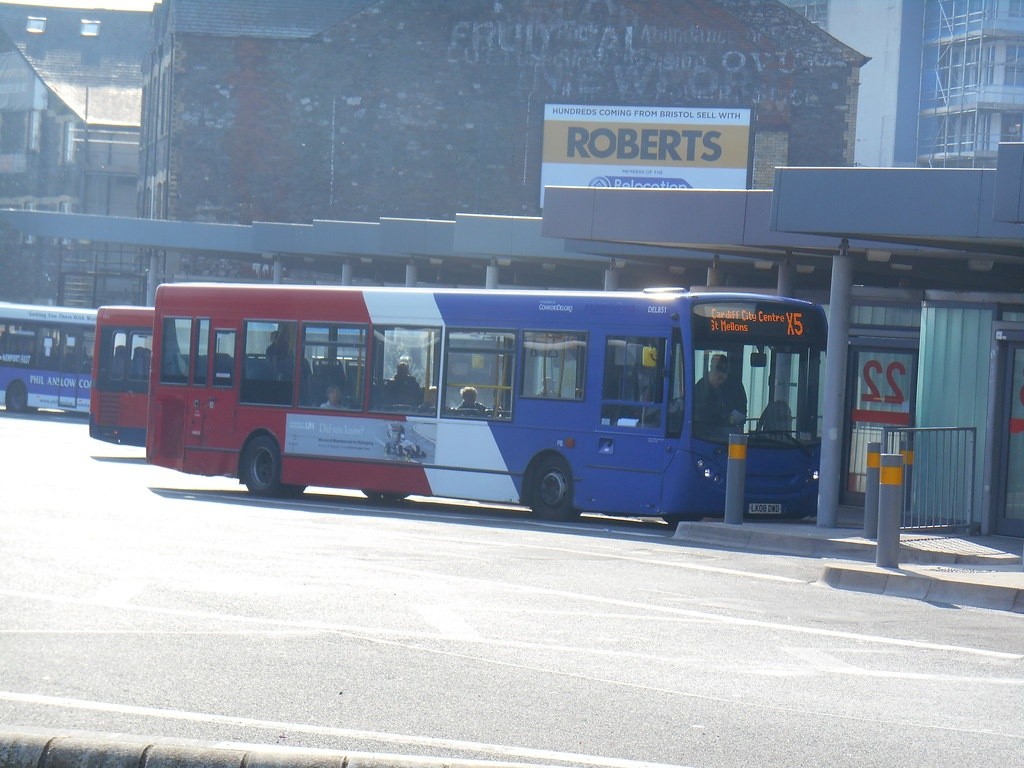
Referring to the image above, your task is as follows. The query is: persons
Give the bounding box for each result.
[386,356,427,407]
[320,385,346,409]
[456,387,500,417]
[694,354,747,441]
[265,322,292,404]
[536,377,559,398]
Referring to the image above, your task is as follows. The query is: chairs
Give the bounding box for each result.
[114,346,151,381]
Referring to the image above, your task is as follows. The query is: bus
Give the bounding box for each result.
[146,284,829,527]
[0,301,643,448]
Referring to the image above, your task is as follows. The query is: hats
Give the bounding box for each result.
[710,355,727,367]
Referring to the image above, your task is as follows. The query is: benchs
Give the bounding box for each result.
[165,351,365,407]
